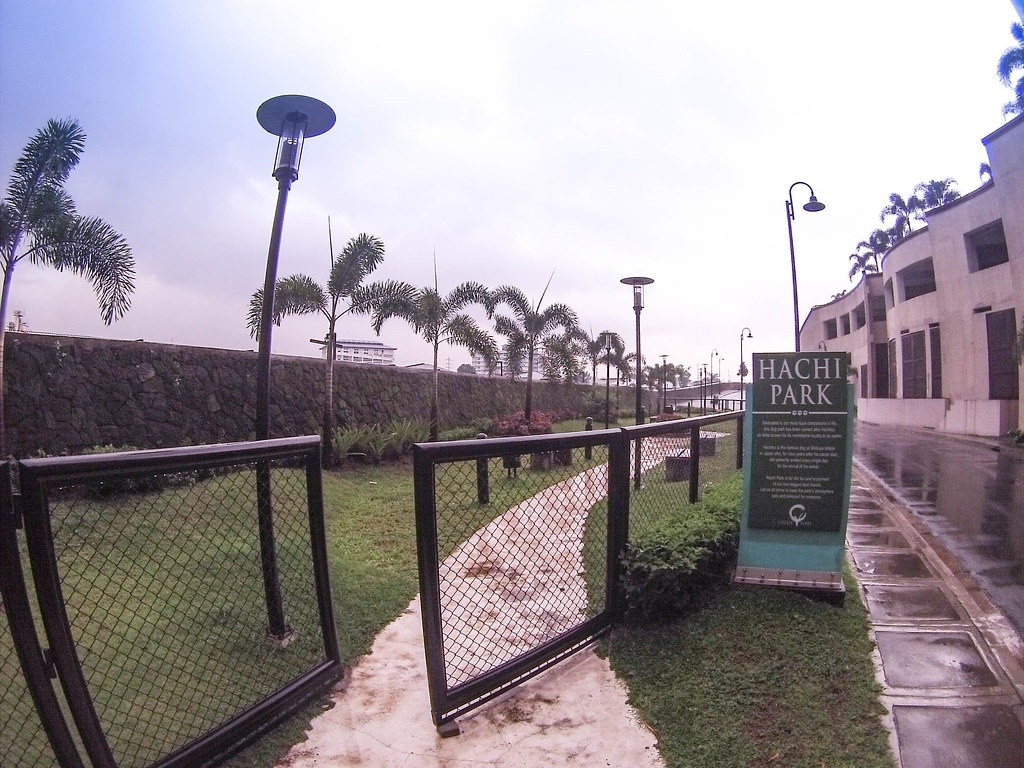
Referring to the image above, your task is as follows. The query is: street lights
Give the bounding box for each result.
[719,353,725,396]
[600,333,618,429]
[659,354,670,412]
[710,349,719,404]
[702,364,708,415]
[740,326,753,409]
[786,182,826,353]
[620,277,656,490]
[252,94,337,637]
